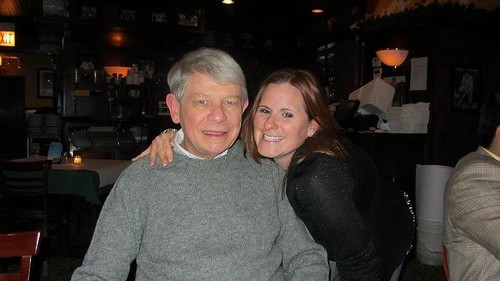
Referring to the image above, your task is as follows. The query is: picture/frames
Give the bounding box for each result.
[36,67,53,98]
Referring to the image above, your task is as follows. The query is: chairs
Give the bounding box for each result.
[0,158,72,281]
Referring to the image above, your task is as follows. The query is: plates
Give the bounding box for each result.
[26,113,62,136]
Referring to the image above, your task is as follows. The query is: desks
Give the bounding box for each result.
[13,157,131,241]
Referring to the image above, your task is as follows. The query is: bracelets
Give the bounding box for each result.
[159,128,178,133]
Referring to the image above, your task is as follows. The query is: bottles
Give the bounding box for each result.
[61,152,68,164]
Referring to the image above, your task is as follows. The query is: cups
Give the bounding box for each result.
[126,63,144,84]
[73,155,81,164]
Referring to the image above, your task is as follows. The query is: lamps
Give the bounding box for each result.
[375,48,409,68]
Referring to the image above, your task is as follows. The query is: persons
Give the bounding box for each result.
[441,98,500,281]
[131,67,417,281]
[388,77,404,107]
[70,47,331,281]
